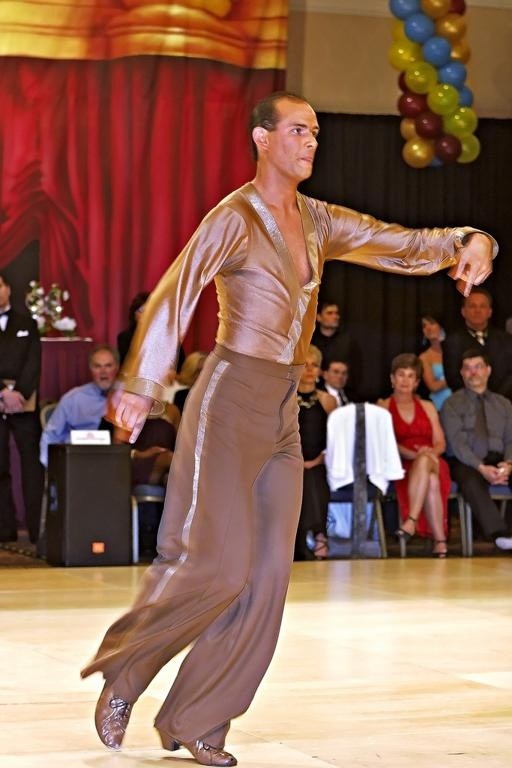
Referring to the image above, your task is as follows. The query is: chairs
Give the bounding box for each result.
[36,403,512,565]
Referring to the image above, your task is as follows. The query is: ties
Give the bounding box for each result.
[475,330,485,346]
[473,395,489,462]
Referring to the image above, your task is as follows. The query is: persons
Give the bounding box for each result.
[292,286,512,558]
[0,277,46,546]
[82,91,500,766]
[38,346,121,465]
[113,292,208,489]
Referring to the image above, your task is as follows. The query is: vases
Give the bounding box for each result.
[27,276,75,333]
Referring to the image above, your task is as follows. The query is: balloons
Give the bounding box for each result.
[388,0,481,169]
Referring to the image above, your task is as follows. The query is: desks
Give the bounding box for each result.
[8,336,96,525]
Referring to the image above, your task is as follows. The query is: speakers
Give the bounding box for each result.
[47,443,133,567]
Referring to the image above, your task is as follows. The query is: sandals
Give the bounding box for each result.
[313,538,329,559]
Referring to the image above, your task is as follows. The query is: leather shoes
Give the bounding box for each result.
[395,517,417,540]
[94,680,133,753]
[431,540,448,559]
[157,729,237,766]
